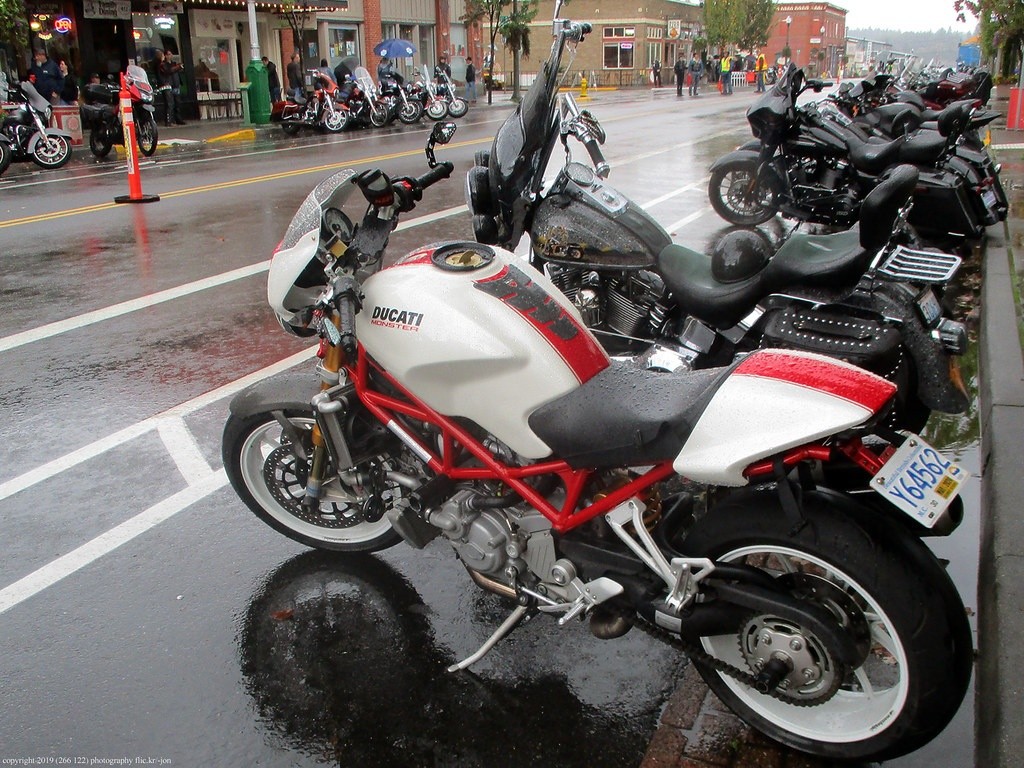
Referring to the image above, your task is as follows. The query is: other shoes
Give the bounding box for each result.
[754,90,763,93]
[170,121,186,127]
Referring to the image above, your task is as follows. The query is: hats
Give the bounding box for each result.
[465,56,472,61]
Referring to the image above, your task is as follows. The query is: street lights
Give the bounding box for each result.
[783,15,793,69]
[818,25,825,73]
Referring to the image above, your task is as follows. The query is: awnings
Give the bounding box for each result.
[183,0,349,12]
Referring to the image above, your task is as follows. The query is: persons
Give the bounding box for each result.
[674,55,688,96]
[975,67,992,106]
[28,48,64,105]
[688,53,704,96]
[334,57,359,90]
[153,49,187,127]
[705,53,758,87]
[83,72,114,122]
[58,59,78,106]
[754,49,767,93]
[465,57,477,102]
[378,58,394,89]
[287,52,304,98]
[719,51,733,95]
[652,59,664,87]
[318,59,337,85]
[262,56,281,104]
[434,56,451,96]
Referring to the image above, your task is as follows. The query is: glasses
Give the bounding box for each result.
[91,76,99,78]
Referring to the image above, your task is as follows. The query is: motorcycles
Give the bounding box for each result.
[463,0,961,420]
[0,75,69,170]
[269,63,468,130]
[78,61,160,154]
[707,63,1008,257]
[217,111,974,764]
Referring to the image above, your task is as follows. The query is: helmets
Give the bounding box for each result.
[439,56,447,62]
[710,229,773,283]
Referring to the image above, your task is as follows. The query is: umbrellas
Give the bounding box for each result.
[374,39,418,58]
[487,43,498,50]
